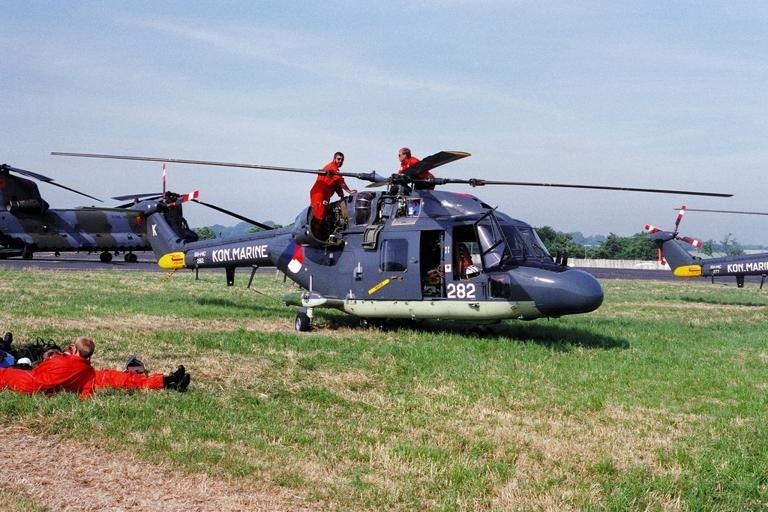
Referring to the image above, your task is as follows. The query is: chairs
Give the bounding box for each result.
[454,243,483,280]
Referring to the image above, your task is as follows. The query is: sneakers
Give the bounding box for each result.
[162,363,191,393]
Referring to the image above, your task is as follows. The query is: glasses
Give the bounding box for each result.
[336,157,344,161]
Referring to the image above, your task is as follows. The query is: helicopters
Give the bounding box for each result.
[50,150,735,332]
[640,205,768,289]
[0,162,274,263]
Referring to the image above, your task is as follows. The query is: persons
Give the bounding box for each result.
[0,335,96,402]
[397,147,437,191]
[44,347,191,393]
[311,150,356,233]
